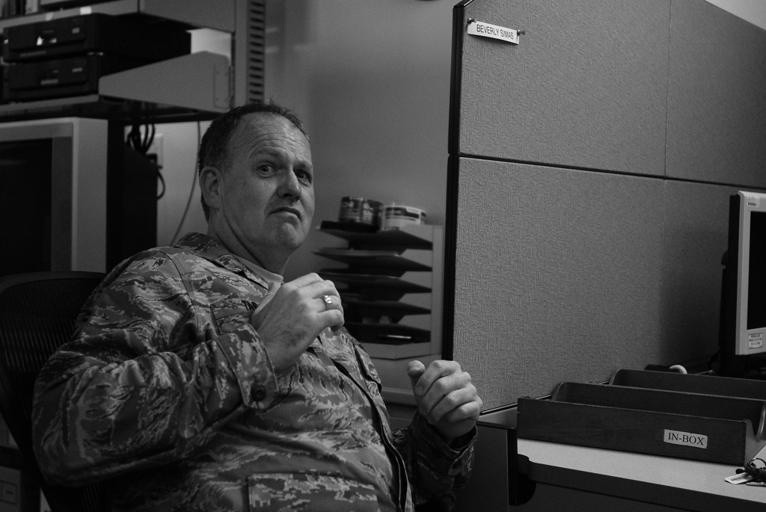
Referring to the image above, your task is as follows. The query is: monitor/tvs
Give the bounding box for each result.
[0,118,158,281]
[719,188,766,361]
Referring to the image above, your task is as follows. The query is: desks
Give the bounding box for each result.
[515,433,764,512]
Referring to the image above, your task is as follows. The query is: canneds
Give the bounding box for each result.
[338,195,428,233]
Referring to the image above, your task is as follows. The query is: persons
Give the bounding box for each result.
[27,97,486,512]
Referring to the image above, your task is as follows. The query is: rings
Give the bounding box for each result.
[321,292,333,310]
